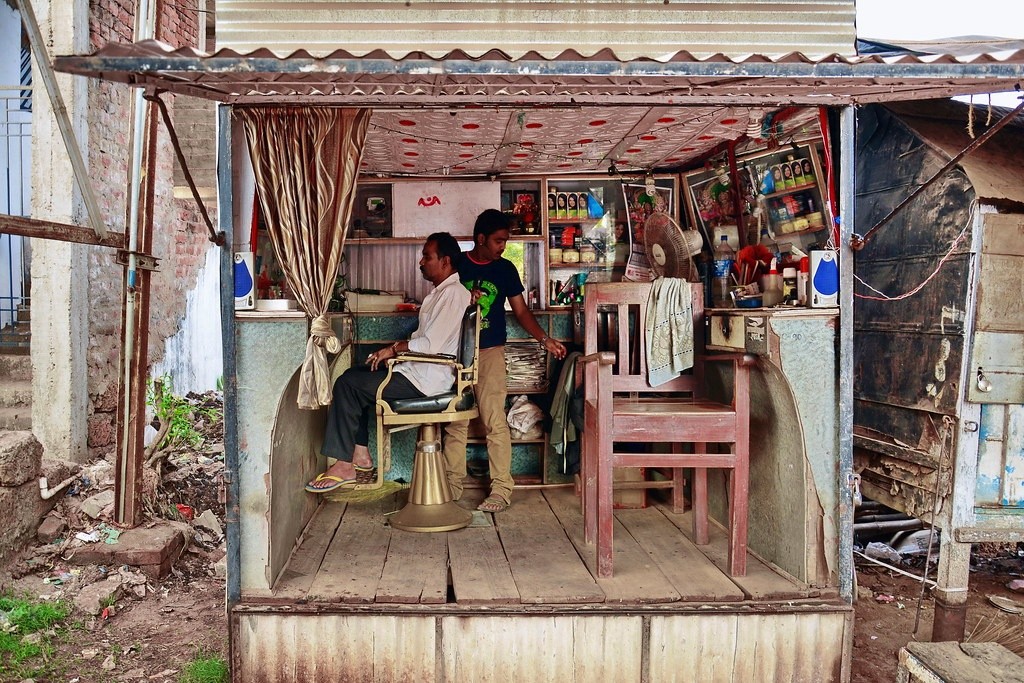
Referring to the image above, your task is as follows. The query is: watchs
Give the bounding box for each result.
[391,342,399,355]
[540,336,550,350]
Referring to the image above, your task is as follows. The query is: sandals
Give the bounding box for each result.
[477,494,507,512]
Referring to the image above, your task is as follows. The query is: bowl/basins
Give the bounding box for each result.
[735,298,761,307]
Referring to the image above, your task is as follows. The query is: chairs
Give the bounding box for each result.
[346,305,482,532]
[578,283,758,580]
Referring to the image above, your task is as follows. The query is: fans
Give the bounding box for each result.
[644,214,701,283]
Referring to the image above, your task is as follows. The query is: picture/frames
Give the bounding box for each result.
[501,141,834,311]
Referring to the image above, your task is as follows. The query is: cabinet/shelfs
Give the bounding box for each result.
[462,309,638,489]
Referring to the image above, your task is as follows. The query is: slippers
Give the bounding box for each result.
[306,473,356,492]
[353,463,374,472]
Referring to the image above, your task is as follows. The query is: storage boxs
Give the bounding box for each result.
[612,467,646,509]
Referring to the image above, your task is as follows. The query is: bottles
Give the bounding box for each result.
[761,228,782,264]
[713,234,734,308]
[762,269,783,307]
[780,266,797,301]
[549,248,596,263]
[775,210,824,234]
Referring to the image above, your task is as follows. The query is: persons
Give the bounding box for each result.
[305,232,472,493]
[615,223,624,241]
[771,159,812,183]
[442,209,566,512]
[548,193,588,210]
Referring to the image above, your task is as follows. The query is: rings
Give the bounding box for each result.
[368,353,374,359]
